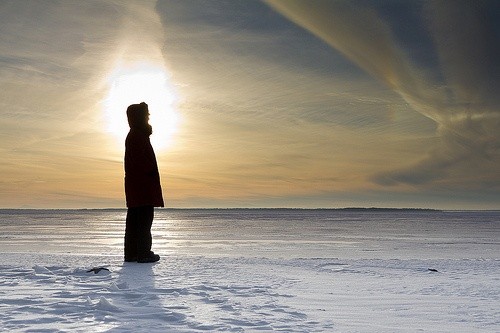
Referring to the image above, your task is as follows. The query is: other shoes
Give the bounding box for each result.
[124,251,160,263]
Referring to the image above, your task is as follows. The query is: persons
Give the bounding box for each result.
[124,102,165,263]
